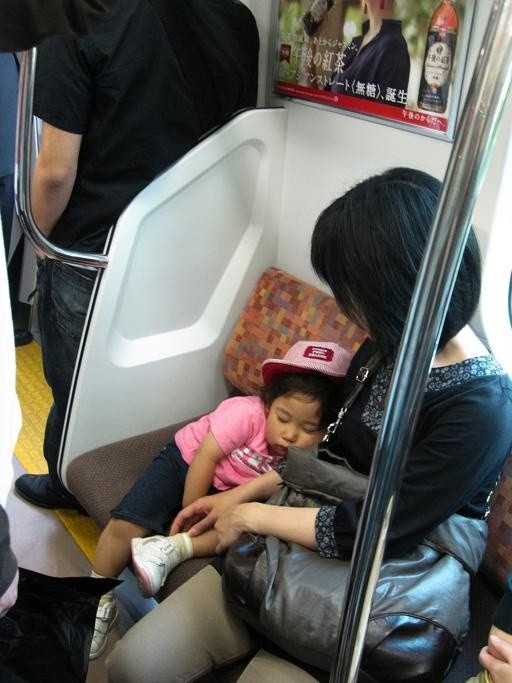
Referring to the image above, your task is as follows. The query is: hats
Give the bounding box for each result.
[261,340,354,385]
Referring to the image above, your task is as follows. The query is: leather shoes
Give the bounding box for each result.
[14,329,33,346]
[15,474,90,517]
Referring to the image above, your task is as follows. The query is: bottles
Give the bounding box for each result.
[298,0,338,37]
[417,0,459,113]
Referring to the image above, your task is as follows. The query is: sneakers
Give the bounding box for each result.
[130,535,180,598]
[89,594,119,661]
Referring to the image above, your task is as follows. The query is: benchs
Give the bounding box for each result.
[63,269,512,683]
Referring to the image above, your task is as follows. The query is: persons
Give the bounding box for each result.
[102,166,511,682]
[10,0,261,521]
[467,582,511,681]
[85,339,354,662]
[327,0,411,104]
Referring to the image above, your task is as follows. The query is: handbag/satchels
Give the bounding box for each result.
[222,440,488,683]
